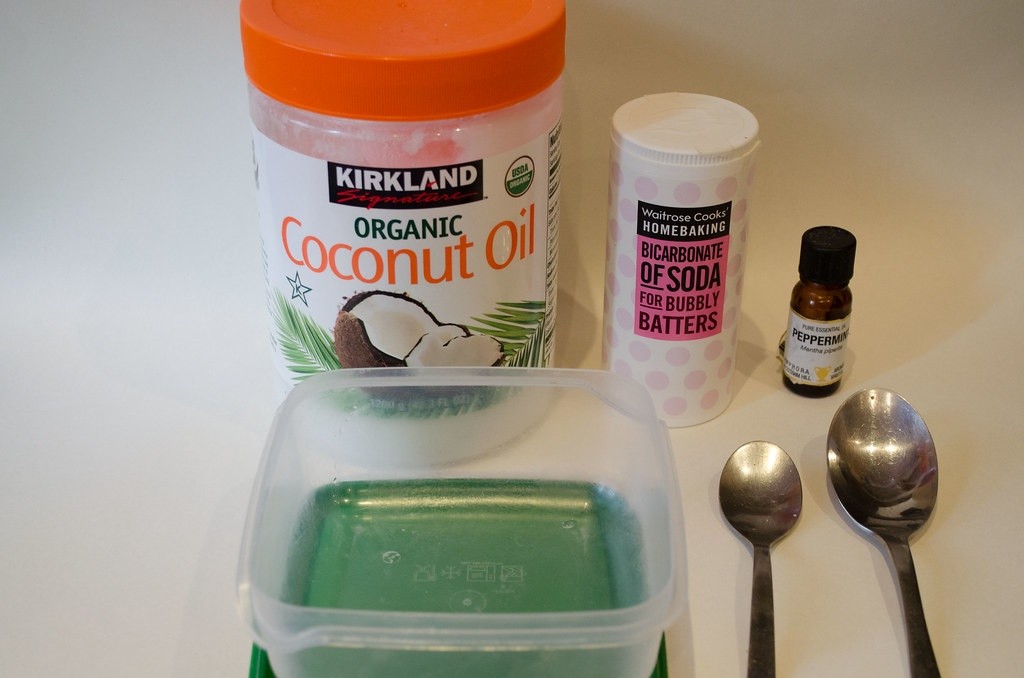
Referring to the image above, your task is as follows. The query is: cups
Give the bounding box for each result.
[600,90,762,429]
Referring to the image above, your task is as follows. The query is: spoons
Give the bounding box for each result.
[826,386,941,678]
[718,440,803,678]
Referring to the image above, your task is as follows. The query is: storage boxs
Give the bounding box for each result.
[235,366,686,678]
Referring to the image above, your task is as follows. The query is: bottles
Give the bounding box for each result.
[780,226,857,400]
[239,0,569,464]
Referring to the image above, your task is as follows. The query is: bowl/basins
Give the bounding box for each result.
[234,366,690,678]
